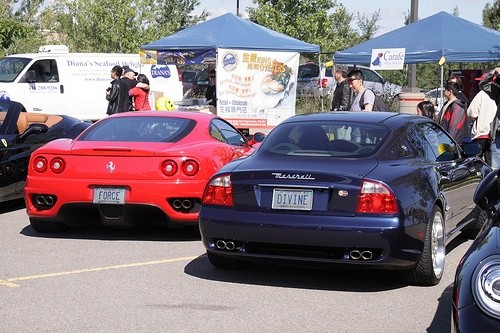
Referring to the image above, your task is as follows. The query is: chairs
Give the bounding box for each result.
[299,126,333,151]
[0,111,63,133]
[29,64,44,81]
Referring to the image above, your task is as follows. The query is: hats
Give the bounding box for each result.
[475,73,493,81]
[124,69,138,76]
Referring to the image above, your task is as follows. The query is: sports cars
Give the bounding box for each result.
[0,98,97,205]
[23,111,267,233]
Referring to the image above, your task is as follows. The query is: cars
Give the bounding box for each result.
[450,169,500,333]
[201,112,492,285]
[182,69,211,95]
[426,88,448,106]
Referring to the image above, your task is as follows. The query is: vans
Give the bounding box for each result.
[0,44,184,122]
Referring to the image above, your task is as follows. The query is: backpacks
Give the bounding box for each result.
[360,88,391,113]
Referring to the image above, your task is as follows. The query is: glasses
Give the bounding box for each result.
[346,78,358,83]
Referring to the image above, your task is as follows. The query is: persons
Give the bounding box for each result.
[206,70,216,105]
[332,69,352,140]
[106,66,151,114]
[347,69,376,143]
[467,67,500,170]
[417,101,434,119]
[439,75,469,144]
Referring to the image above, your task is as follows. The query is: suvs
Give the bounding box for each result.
[296,62,403,112]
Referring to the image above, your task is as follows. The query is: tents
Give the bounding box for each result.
[140,12,323,111]
[335,10,500,110]
